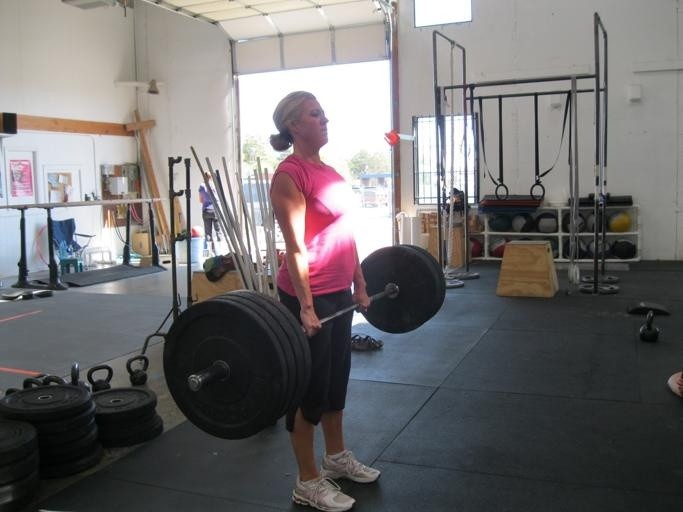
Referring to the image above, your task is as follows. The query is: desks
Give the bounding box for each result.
[0,198,162,291]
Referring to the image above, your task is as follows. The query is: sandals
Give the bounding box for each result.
[351,334,383,350]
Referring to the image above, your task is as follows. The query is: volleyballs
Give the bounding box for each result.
[470,211,637,259]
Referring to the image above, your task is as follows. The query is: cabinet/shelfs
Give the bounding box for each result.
[417,205,642,262]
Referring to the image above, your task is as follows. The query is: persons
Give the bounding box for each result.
[269,91,384,512]
[200,171,225,243]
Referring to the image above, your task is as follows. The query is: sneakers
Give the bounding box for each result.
[321,450,381,484]
[291,473,355,512]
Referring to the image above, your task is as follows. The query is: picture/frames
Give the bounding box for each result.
[4,147,39,205]
[44,164,84,204]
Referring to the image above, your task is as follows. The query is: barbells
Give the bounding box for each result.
[163,245,445,440]
